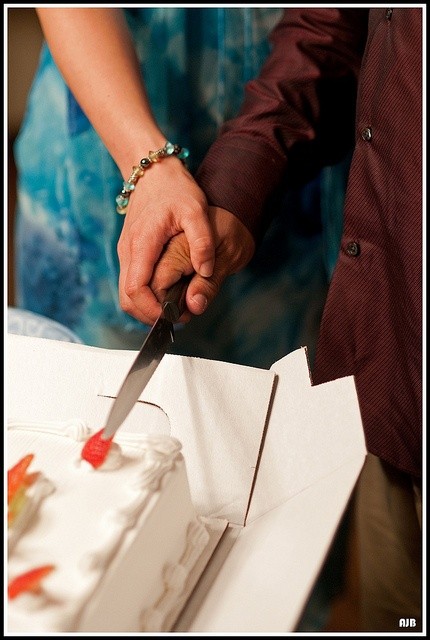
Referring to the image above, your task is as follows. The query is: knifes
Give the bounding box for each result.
[99,280,189,444]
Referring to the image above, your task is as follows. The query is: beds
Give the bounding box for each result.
[5,332,370,633]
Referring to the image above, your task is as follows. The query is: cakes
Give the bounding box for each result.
[7,427,212,633]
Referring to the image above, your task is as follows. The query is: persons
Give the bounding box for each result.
[150,2,426,486]
[14,7,346,368]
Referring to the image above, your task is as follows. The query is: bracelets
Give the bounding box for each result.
[116,143,193,214]
